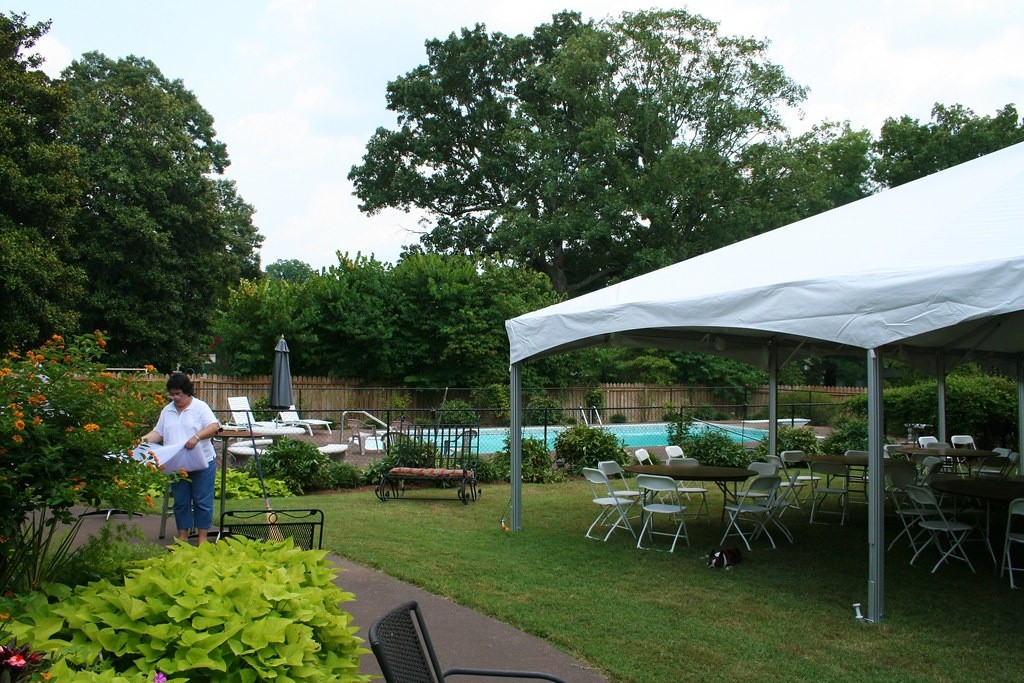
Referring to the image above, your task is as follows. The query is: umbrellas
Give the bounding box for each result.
[268,334,295,429]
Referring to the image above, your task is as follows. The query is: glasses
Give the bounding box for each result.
[167,392,182,400]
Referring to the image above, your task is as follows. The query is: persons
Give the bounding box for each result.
[137,373,219,546]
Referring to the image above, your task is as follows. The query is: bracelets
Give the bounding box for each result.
[194,434,200,441]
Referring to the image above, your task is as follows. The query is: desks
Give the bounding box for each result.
[928,480,1024,564]
[624,465,759,538]
[188,431,263,543]
[799,457,868,505]
[76,423,150,521]
[895,448,1001,480]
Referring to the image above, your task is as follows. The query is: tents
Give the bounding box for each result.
[505,141,1024,625]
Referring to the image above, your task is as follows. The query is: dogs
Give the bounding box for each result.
[707,546,743,571]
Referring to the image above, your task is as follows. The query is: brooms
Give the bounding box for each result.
[246,410,285,543]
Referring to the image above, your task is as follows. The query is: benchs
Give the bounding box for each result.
[232,439,349,469]
[375,415,482,506]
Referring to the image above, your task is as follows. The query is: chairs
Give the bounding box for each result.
[212,396,421,455]
[220,509,324,550]
[883,435,1024,590]
[583,446,869,554]
[368,601,573,683]
